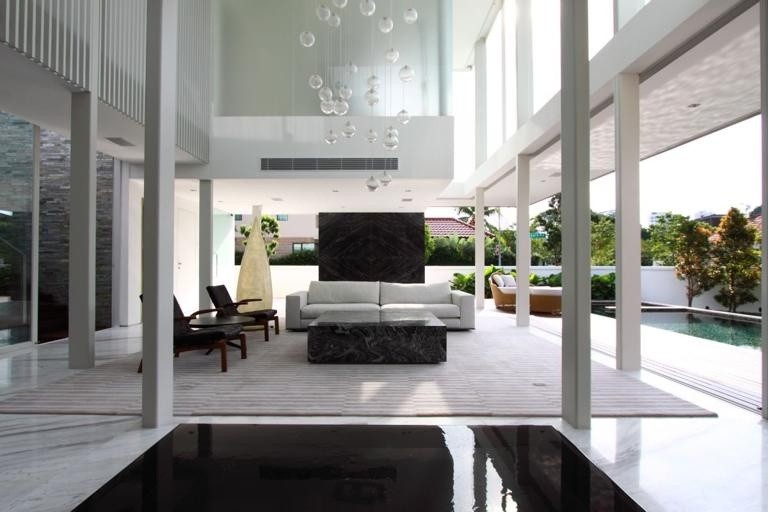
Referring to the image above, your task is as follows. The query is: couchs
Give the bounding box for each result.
[286,281,475,333]
[488,268,563,316]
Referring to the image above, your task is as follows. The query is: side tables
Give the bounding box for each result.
[190,313,256,356]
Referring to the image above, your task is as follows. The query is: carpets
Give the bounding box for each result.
[0,329,719,417]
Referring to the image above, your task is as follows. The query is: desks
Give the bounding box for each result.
[70,423,645,512]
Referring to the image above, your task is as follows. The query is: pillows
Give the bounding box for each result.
[491,276,504,289]
[500,274,516,290]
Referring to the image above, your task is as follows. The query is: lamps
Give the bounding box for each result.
[298,0,418,193]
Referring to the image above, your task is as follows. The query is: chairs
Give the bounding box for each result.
[207,285,278,342]
[138,290,247,371]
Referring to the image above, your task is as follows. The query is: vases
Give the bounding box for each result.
[237,205,273,330]
